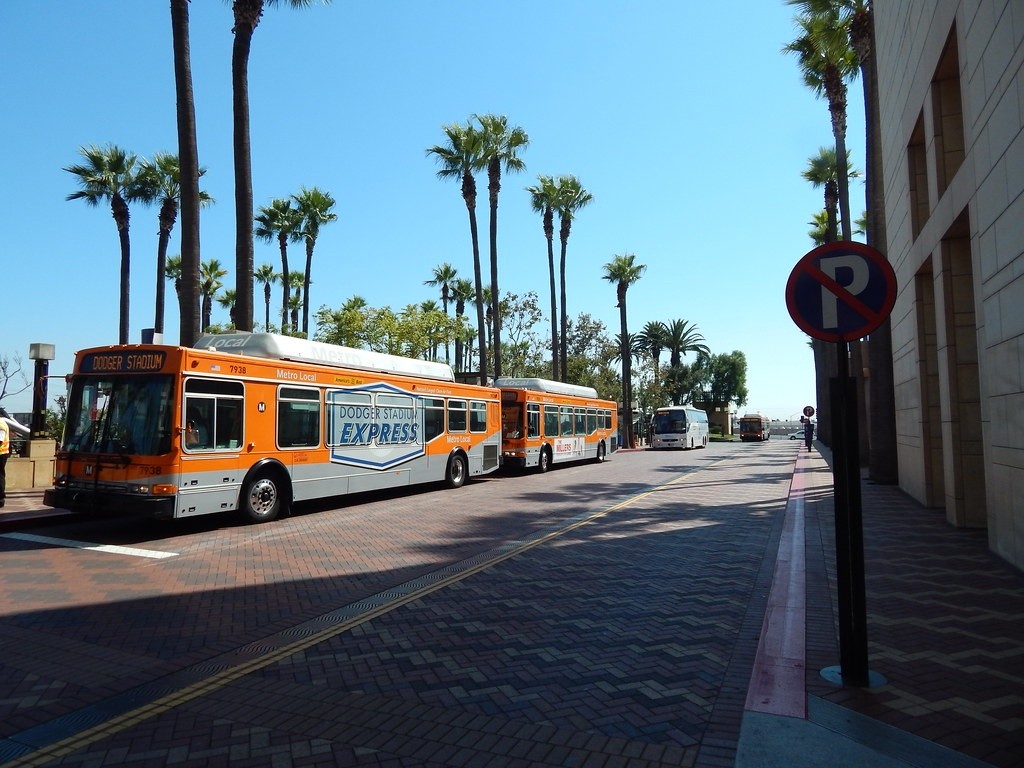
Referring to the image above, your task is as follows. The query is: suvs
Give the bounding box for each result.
[788,430,816,439]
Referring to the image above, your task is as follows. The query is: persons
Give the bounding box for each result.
[184,406,208,449]
[0,418,9,508]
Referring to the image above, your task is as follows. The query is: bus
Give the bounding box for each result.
[737,414,771,442]
[650,405,709,450]
[39,332,502,522]
[494,377,618,473]
